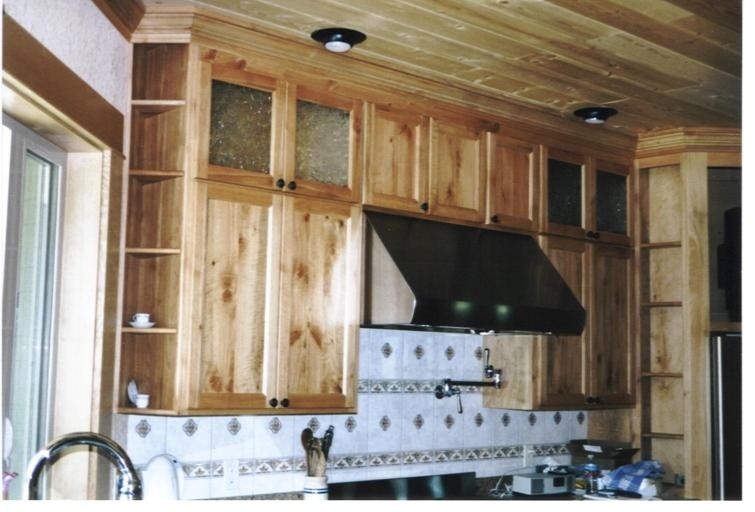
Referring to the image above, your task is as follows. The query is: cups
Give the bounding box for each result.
[132,313,150,324]
[136,394,149,409]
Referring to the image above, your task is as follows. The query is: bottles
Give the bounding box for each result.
[303,473,329,502]
[585,472,594,494]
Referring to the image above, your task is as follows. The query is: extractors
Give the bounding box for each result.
[359,205,585,336]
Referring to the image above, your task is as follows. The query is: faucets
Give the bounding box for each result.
[19,430,142,500]
[433,380,464,414]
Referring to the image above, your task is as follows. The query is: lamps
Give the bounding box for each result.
[311,27,366,54]
[574,107,617,125]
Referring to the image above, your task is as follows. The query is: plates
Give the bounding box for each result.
[128,379,140,404]
[126,321,156,329]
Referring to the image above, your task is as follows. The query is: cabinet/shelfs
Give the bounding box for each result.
[106,13,364,418]
[364,59,541,236]
[482,110,635,411]
